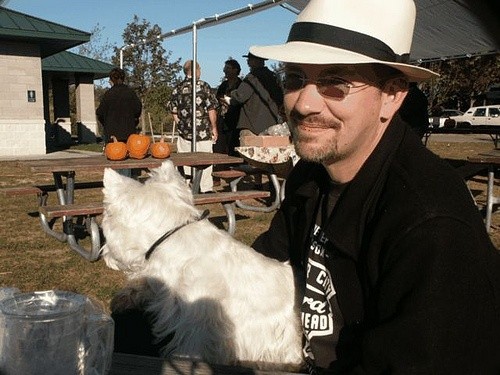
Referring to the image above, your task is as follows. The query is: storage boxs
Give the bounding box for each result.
[239,135,291,148]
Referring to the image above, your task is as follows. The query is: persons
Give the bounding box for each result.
[169,60,219,194]
[95,68,142,180]
[249,0,500,375]
[215,60,253,181]
[220,52,285,191]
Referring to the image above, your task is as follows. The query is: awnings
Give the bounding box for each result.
[279,0,500,65]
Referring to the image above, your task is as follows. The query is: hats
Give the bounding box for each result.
[248,0,441,82]
[242,52,269,61]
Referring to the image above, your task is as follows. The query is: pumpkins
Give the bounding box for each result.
[151,137,170,159]
[106,135,128,160]
[127,132,151,159]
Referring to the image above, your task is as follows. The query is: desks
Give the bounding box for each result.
[466,147,500,234]
[20,151,244,261]
[235,146,301,214]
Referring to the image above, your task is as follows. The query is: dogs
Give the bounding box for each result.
[99,160,304,371]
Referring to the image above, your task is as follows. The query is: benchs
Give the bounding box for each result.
[0,174,193,241]
[38,190,271,262]
[211,169,281,213]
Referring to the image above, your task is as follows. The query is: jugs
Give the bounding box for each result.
[0,290,115,375]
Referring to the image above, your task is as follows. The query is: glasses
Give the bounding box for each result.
[283,76,385,101]
